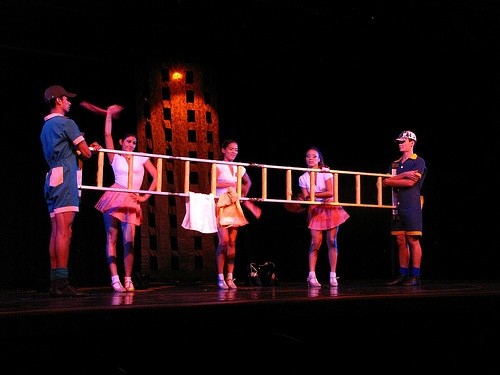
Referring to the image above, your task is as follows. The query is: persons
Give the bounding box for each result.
[40,85,103,298]
[298,148,350,286]
[95,104,158,292]
[382,130,427,286]
[214,138,251,289]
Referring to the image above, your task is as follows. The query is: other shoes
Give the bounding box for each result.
[50,277,83,297]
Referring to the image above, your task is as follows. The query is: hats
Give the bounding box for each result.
[394,131,416,142]
[44,86,76,100]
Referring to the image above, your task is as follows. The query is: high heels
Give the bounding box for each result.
[226,278,237,289]
[217,280,229,289]
[330,277,339,286]
[307,276,321,287]
[124,281,135,291]
[111,282,126,292]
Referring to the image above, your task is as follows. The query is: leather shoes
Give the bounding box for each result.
[402,274,420,286]
[387,275,408,286]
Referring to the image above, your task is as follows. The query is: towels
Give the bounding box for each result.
[180,191,218,234]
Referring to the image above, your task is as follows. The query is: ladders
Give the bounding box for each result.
[77,147,397,208]
